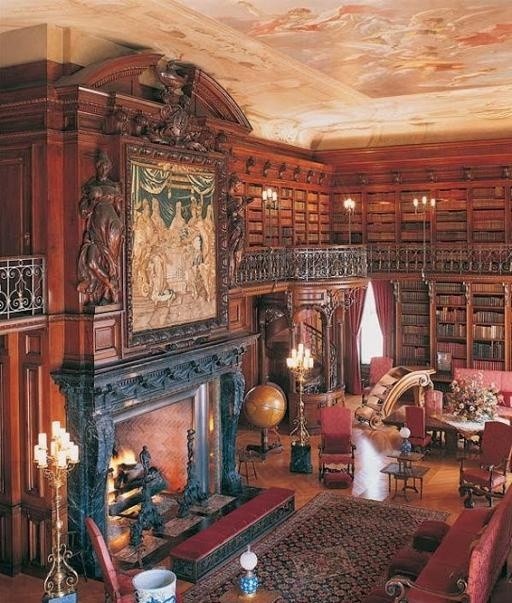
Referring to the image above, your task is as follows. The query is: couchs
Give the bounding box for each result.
[445,366,512,424]
[385,481,512,603]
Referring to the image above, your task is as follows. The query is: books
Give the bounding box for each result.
[233,177,505,243]
[398,279,505,371]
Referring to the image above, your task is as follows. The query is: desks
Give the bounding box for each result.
[381,411,511,450]
[380,449,430,503]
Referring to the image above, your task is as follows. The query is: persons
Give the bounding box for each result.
[77,146,124,305]
[226,171,254,283]
[132,194,216,306]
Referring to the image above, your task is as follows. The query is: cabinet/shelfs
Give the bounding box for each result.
[392,282,512,401]
[232,173,512,274]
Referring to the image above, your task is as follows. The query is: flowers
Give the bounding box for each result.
[441,370,504,424]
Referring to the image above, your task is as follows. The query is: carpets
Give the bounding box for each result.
[177,491,452,603]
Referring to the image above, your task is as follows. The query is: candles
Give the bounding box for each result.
[286,343,314,369]
[34,421,80,468]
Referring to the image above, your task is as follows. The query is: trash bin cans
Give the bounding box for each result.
[132,568,177,603]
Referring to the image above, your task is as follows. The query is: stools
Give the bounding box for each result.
[323,472,353,489]
[365,520,450,603]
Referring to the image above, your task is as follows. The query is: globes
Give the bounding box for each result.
[243,382,288,458]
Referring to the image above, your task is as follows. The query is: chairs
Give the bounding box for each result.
[316,406,356,482]
[84,517,146,603]
[361,357,394,407]
[404,407,432,461]
[456,421,511,501]
